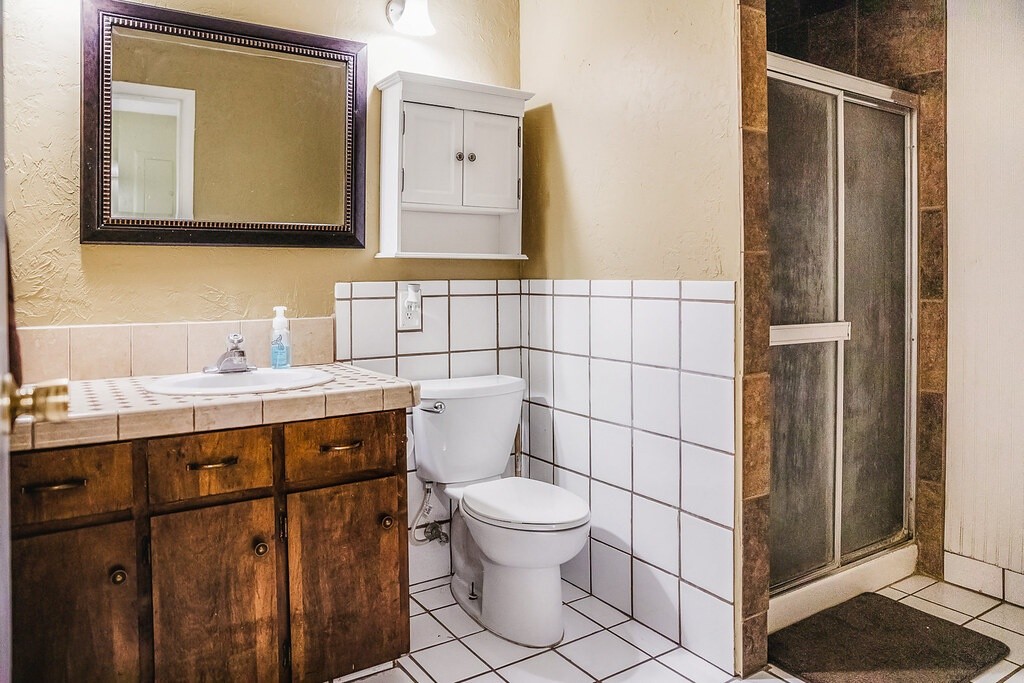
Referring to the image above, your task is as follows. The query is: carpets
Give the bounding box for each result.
[767,592,1008,682]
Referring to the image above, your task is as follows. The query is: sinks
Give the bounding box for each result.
[145,367,335,394]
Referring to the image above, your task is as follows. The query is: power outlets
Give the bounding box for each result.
[398,291,423,331]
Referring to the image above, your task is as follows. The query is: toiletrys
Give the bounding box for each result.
[270,306,292,369]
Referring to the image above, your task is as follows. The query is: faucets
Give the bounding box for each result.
[202,333,257,374]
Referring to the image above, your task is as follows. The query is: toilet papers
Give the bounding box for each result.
[406,426,415,457]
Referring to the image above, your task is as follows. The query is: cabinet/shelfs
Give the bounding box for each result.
[8,411,415,682]
[376,70,533,262]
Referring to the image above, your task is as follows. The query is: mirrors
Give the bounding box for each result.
[82,1,367,247]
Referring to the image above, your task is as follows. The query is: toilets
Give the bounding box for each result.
[410,375,590,650]
